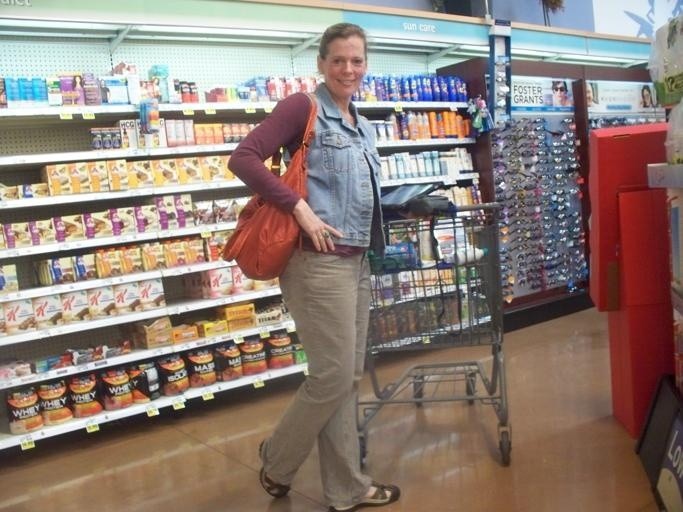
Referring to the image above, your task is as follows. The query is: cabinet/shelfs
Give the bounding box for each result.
[0,98,493,452]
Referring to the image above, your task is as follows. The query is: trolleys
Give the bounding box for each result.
[353,198,515,468]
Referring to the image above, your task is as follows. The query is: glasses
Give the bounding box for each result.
[589,117,668,130]
[491,54,588,303]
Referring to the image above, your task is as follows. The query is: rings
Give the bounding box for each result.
[323,231,329,238]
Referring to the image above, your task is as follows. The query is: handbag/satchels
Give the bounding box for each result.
[222,146,313,280]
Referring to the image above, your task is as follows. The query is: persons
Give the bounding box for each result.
[227,24,400,511]
[586,82,599,110]
[552,81,575,106]
[636,86,656,109]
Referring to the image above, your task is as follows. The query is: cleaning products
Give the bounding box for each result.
[452,248,489,264]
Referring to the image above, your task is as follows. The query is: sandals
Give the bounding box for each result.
[330,481,400,512]
[258,439,290,498]
